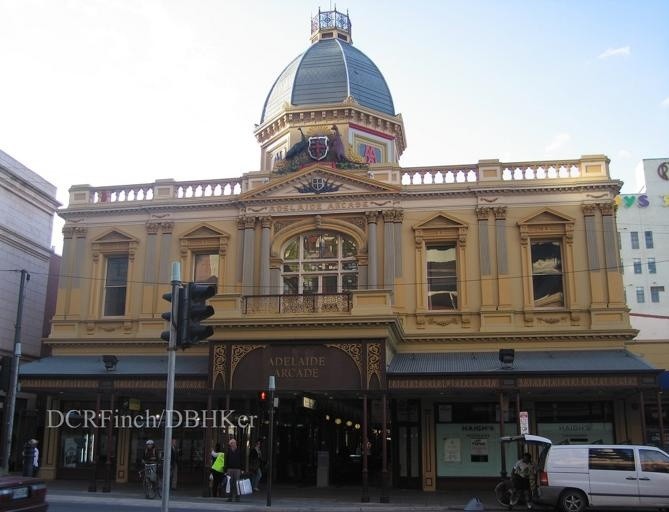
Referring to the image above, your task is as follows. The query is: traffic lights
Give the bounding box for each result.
[182,278,218,349]
[257,389,269,403]
[156,283,182,352]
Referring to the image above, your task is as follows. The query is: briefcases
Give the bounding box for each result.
[236,478,253,496]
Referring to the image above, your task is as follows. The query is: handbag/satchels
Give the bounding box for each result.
[221,475,231,494]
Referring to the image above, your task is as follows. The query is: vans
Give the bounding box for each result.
[498,430,668,512]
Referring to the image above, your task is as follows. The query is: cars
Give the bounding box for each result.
[0,466,49,511]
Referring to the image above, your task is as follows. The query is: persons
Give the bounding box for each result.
[170,438,181,490]
[210,448,227,497]
[249,439,265,492]
[507,453,535,511]
[140,439,162,498]
[223,438,245,502]
[22,438,39,478]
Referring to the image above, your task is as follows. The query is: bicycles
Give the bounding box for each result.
[139,458,162,501]
[494,468,544,508]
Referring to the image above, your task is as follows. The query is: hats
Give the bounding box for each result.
[145,440,154,445]
[29,439,38,444]
